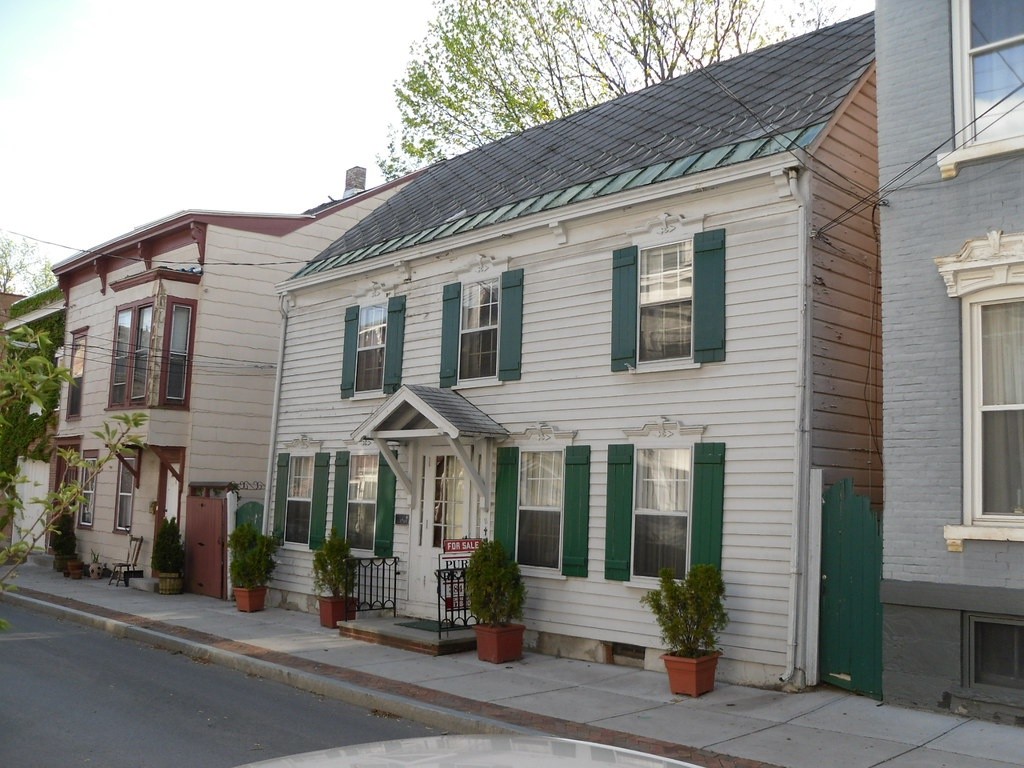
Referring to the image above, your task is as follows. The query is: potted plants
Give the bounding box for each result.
[311,525,358,630]
[224,520,283,613]
[150,516,186,595]
[637,562,730,698]
[464,539,530,665]
[89,548,103,579]
[49,511,79,571]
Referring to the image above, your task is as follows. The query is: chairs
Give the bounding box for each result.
[108,535,144,587]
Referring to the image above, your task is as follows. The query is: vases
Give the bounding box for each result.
[67,559,84,579]
